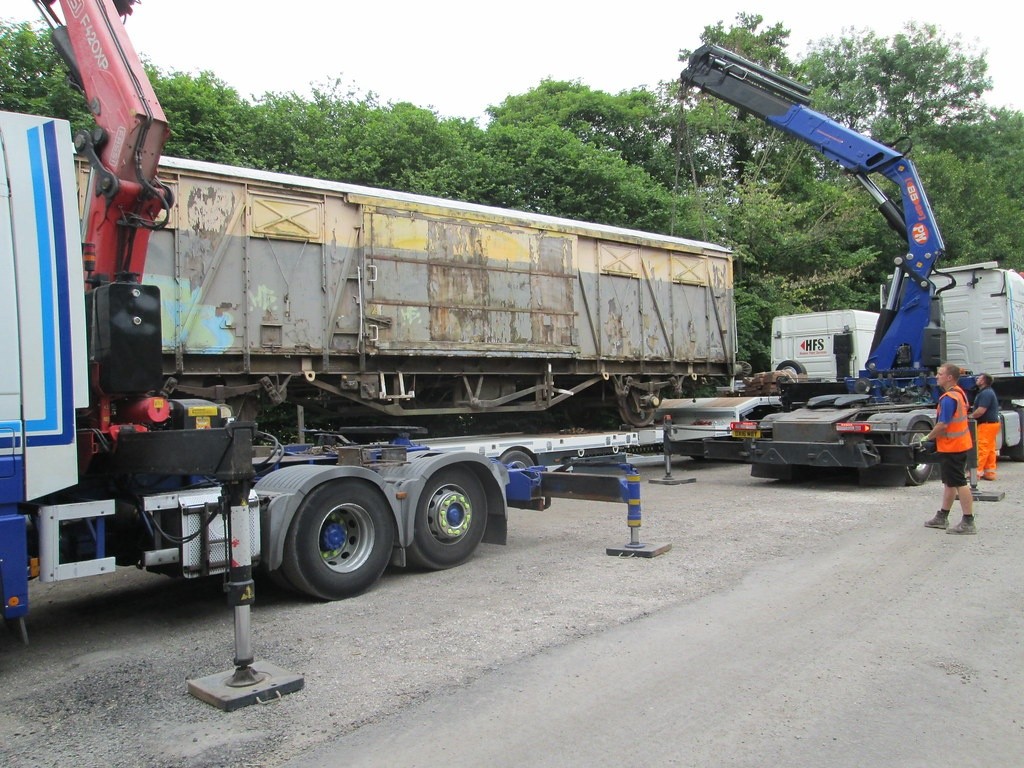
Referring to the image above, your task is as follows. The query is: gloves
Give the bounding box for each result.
[920,436,930,449]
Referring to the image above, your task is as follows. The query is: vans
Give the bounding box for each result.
[770,309,879,376]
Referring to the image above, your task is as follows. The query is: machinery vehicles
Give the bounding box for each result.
[0,0,739,624]
[681,43,1024,486]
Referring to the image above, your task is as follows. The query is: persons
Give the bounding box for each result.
[920,363,977,535]
[968,373,1000,480]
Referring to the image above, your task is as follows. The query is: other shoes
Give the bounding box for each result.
[946,516,977,535]
[925,511,949,528]
